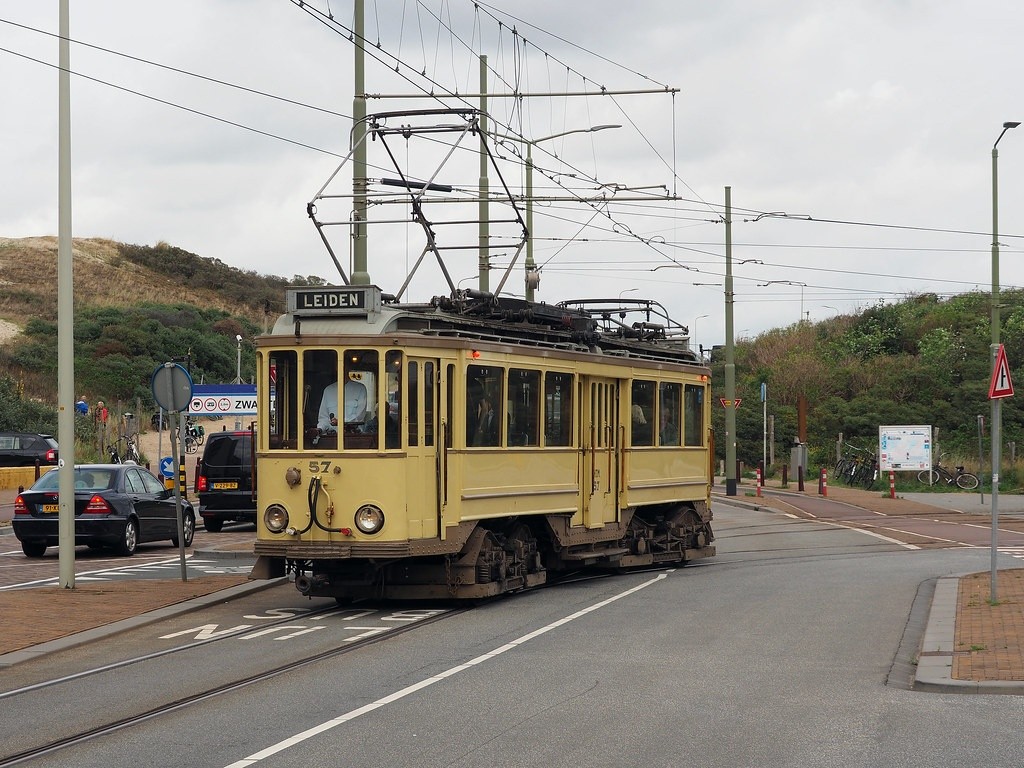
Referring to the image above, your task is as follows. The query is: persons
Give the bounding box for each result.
[631,405,653,446]
[316,360,368,434]
[74,395,89,415]
[365,401,397,434]
[659,408,678,446]
[82,473,95,488]
[95,401,108,426]
[466,372,488,447]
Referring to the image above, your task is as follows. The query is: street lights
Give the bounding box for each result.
[436,123,624,302]
[992,121,1021,606]
[695,315,710,353]
[619,288,639,324]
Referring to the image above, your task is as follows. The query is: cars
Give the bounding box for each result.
[194,431,256,532]
[10,464,197,556]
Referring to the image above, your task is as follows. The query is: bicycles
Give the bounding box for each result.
[918,453,978,490]
[170,419,204,455]
[105,432,141,464]
[834,442,878,490]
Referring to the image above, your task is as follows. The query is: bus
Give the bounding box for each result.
[248,286,718,598]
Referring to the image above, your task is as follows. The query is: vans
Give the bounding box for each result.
[0,432,58,467]
